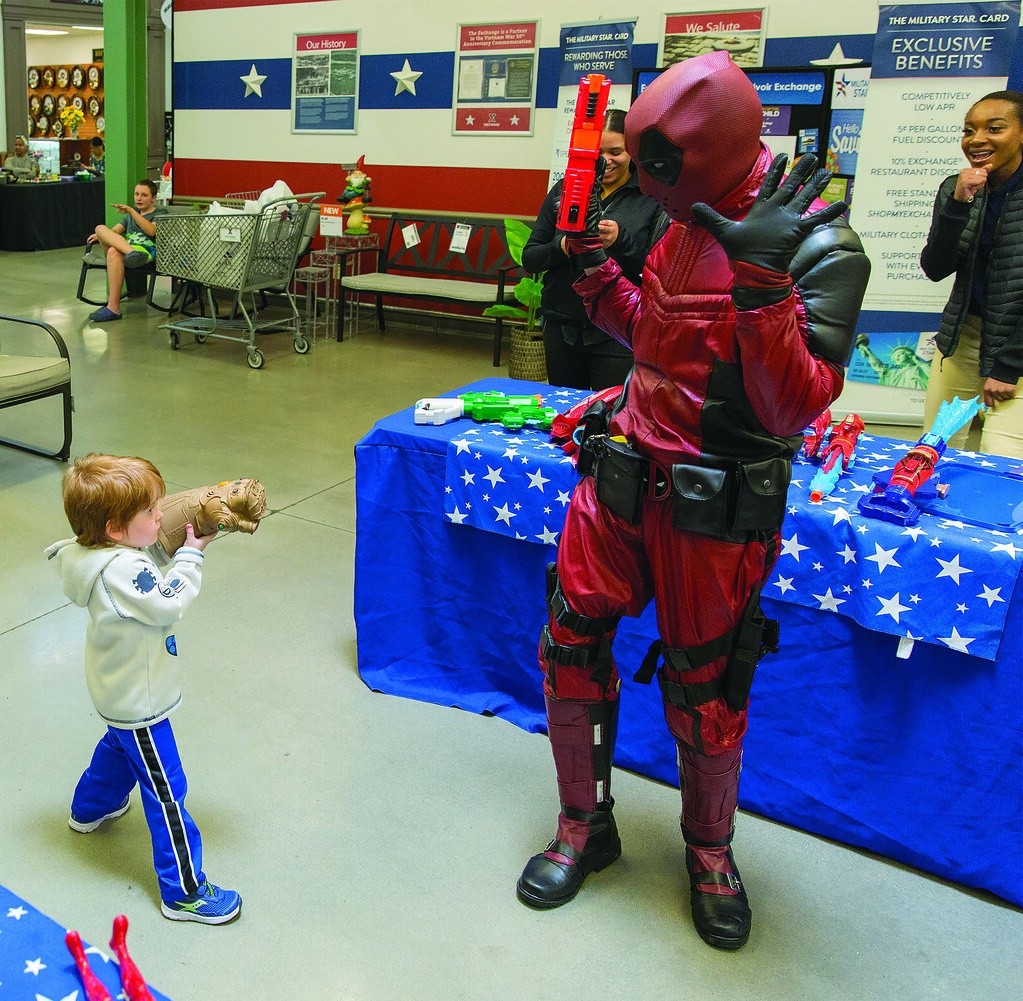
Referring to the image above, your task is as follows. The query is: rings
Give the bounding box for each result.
[975,170,980,174]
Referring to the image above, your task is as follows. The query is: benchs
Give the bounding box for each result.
[0,317,76,462]
[333,211,546,367]
[76,207,319,320]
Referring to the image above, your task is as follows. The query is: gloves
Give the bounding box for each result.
[690,152,849,276]
[564,156,607,271]
[155,477,268,558]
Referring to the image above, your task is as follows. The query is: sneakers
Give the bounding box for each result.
[161,878,243,925]
[67,792,131,834]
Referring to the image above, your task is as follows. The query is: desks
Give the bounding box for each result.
[293,229,380,344]
[354,375,1023,920]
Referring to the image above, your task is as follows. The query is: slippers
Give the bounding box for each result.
[124,251,148,269]
[89,307,122,322]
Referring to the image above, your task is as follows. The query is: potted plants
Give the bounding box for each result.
[482,218,549,382]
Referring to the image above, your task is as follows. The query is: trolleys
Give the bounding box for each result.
[155,191,326,369]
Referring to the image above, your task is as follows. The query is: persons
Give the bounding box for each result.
[857,332,932,390]
[336,153,372,203]
[3,133,40,178]
[515,49,872,952]
[43,451,268,927]
[522,108,669,392]
[87,178,170,321]
[487,62,503,74]
[919,88,1023,460]
[81,135,105,178]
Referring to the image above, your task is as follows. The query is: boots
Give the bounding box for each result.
[516,693,621,910]
[676,742,752,951]
